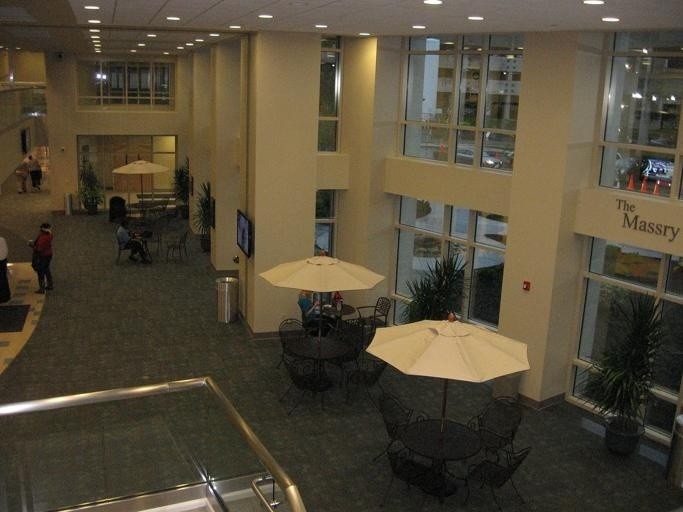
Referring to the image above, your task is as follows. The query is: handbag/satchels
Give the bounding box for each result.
[31,251,40,271]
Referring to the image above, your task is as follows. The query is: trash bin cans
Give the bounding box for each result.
[215,277,239,324]
[666,415,683,488]
[64,192,72,216]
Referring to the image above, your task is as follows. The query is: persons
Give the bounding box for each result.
[27,155,42,192]
[15,162,29,194]
[298,290,336,337]
[0,236,11,304]
[116,220,151,264]
[28,222,54,293]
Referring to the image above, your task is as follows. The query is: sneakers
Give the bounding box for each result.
[18,188,27,194]
[31,185,40,192]
[128,255,154,264]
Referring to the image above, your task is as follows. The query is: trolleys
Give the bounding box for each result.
[14,167,27,195]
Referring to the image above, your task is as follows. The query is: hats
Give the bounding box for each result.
[39,223,52,231]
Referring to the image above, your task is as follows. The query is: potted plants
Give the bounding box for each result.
[174,157,189,218]
[77,160,103,214]
[191,181,214,252]
[573,287,676,457]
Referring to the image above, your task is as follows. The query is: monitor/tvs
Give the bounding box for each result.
[237,209,252,257]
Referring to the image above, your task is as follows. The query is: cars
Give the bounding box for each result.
[429,142,503,169]
[487,146,513,164]
[648,137,675,148]
[616,150,668,175]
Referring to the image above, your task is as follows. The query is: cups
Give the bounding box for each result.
[27,239,35,247]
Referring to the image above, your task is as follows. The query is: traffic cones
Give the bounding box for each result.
[651,179,662,195]
[625,172,635,190]
[639,174,648,192]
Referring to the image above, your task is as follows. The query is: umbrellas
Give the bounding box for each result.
[365,313,531,432]
[258,255,386,376]
[113,159,170,212]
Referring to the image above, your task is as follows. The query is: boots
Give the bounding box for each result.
[45,280,52,290]
[34,280,45,294]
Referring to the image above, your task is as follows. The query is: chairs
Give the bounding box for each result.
[278,296,390,416]
[113,195,190,264]
[373,391,534,512]
